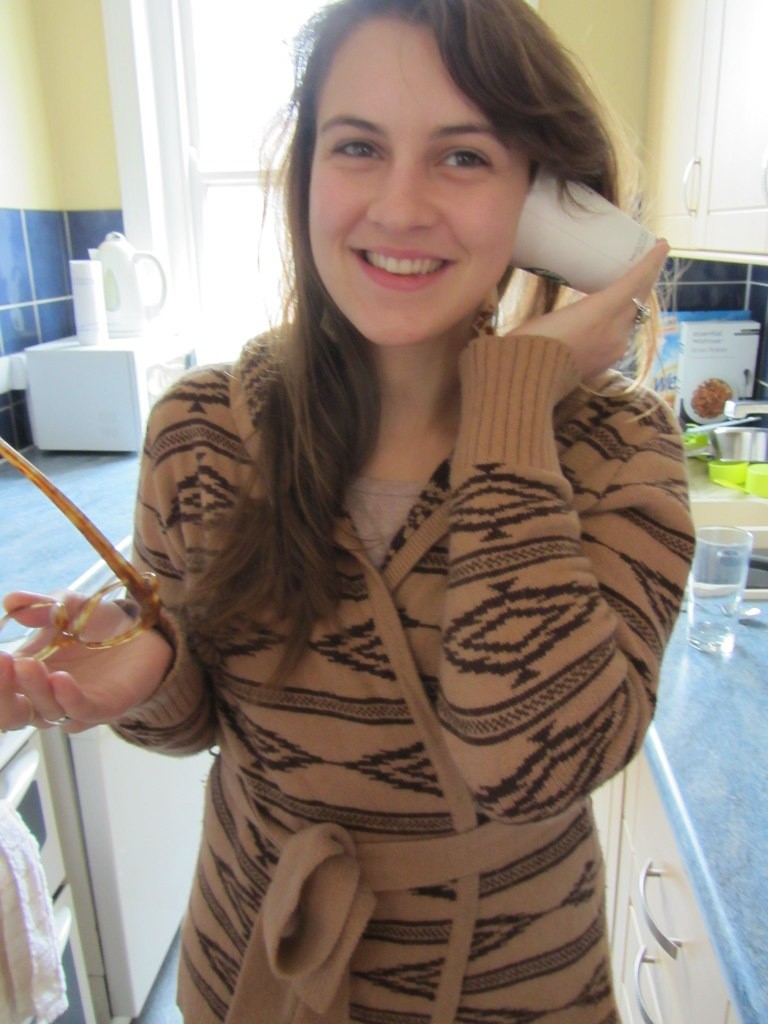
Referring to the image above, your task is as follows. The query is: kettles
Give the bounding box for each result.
[86,232,168,335]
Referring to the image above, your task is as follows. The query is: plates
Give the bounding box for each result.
[683,377,737,425]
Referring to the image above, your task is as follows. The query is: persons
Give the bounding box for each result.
[0,0,695,1022]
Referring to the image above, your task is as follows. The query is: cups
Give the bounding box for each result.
[508,163,659,295]
[686,526,754,653]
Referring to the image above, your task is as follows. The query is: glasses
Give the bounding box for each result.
[0,439,163,662]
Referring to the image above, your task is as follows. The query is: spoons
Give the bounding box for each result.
[681,602,761,619]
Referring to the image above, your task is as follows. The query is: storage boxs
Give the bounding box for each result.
[636,310,763,431]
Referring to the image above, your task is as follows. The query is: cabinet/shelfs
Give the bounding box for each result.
[591,584,767,1024]
[632,0,768,268]
[26,339,210,454]
